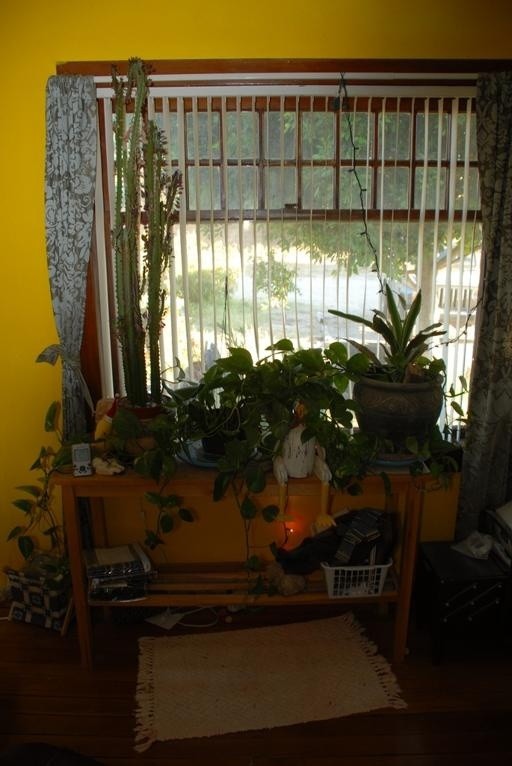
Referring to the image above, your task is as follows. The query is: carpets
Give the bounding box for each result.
[133,612,407,751]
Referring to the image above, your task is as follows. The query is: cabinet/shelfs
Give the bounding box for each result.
[61,468,420,671]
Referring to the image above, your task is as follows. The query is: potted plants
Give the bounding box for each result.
[325,291,465,444]
